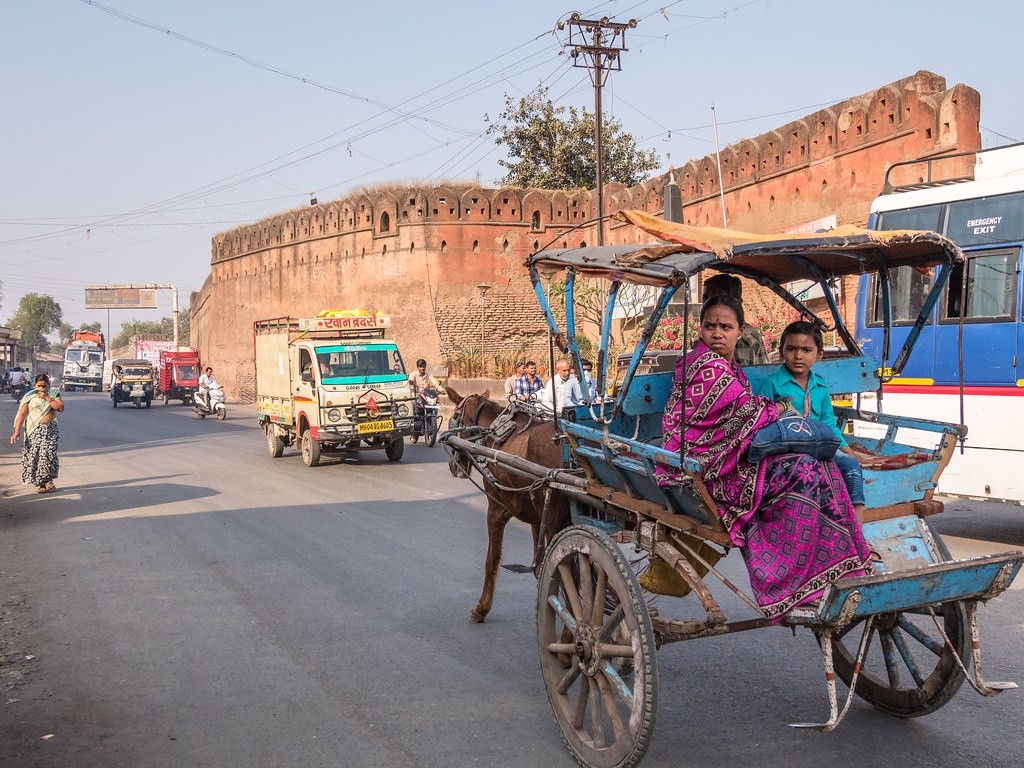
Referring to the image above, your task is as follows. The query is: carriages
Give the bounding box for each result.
[436,208,1024,768]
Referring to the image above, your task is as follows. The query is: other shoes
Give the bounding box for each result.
[38,484,47,493]
[866,540,884,563]
[47,484,56,493]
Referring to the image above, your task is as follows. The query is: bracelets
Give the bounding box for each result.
[775,400,791,412]
[15,429,20,434]
[48,398,54,404]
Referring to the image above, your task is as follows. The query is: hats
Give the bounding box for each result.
[515,361,524,370]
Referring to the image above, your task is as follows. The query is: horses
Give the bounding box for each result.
[445,387,660,662]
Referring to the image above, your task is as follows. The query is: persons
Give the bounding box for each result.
[702,273,771,366]
[394,364,401,372]
[655,294,875,625]
[178,368,194,379]
[199,367,224,406]
[302,354,334,375]
[409,359,446,397]
[504,358,608,414]
[10,367,34,391]
[759,321,882,562]
[10,373,64,493]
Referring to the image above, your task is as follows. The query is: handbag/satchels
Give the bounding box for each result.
[744,408,843,465]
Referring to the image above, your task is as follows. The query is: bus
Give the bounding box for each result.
[852,120,1024,505]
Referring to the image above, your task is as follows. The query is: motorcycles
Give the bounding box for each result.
[409,379,444,448]
[109,359,155,410]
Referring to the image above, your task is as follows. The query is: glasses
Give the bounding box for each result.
[583,369,591,372]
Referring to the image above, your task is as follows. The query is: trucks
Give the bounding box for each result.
[60,339,106,393]
[253,316,420,467]
[157,347,203,406]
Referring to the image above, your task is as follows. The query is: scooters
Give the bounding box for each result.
[191,382,227,421]
[0,376,35,404]
[152,374,161,400]
